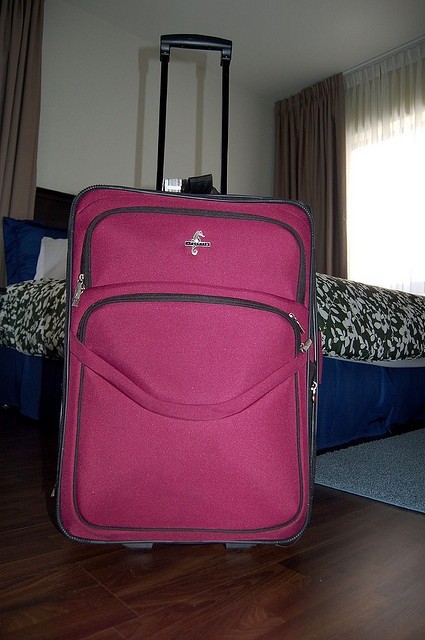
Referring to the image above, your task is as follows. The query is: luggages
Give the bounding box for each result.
[52,28,326,556]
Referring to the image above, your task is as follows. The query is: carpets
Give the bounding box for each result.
[314,426,424,513]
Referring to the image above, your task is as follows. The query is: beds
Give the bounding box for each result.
[0,186,424,453]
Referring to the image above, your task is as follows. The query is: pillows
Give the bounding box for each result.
[4,217,70,286]
[33,236,68,280]
